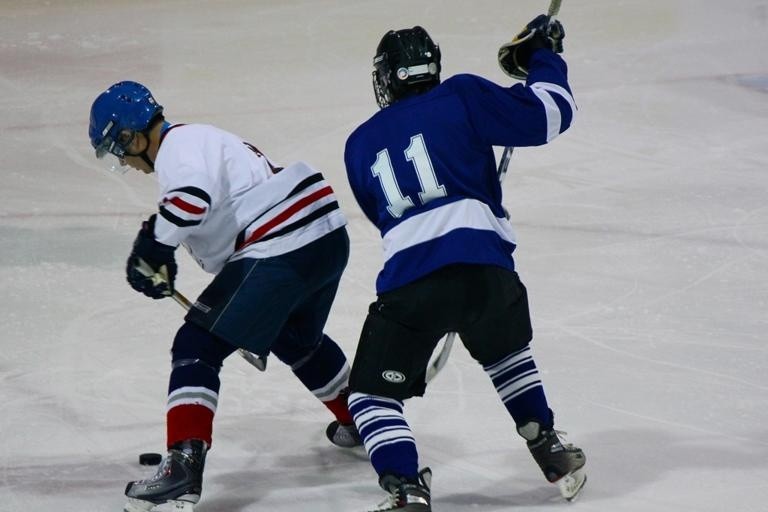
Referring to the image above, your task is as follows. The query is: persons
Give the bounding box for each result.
[89,81,364,504]
[345,14,587,512]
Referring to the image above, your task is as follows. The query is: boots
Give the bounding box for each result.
[326,419,365,449]
[364,467,434,512]
[520,416,588,483]
[123,438,208,504]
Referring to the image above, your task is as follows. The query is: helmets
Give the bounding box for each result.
[88,79,165,160]
[372,25,440,101]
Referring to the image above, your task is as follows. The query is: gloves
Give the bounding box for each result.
[124,213,180,301]
[497,13,567,81]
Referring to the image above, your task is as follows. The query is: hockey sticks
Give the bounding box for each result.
[153,278,267,370]
[425,0,561,382]
